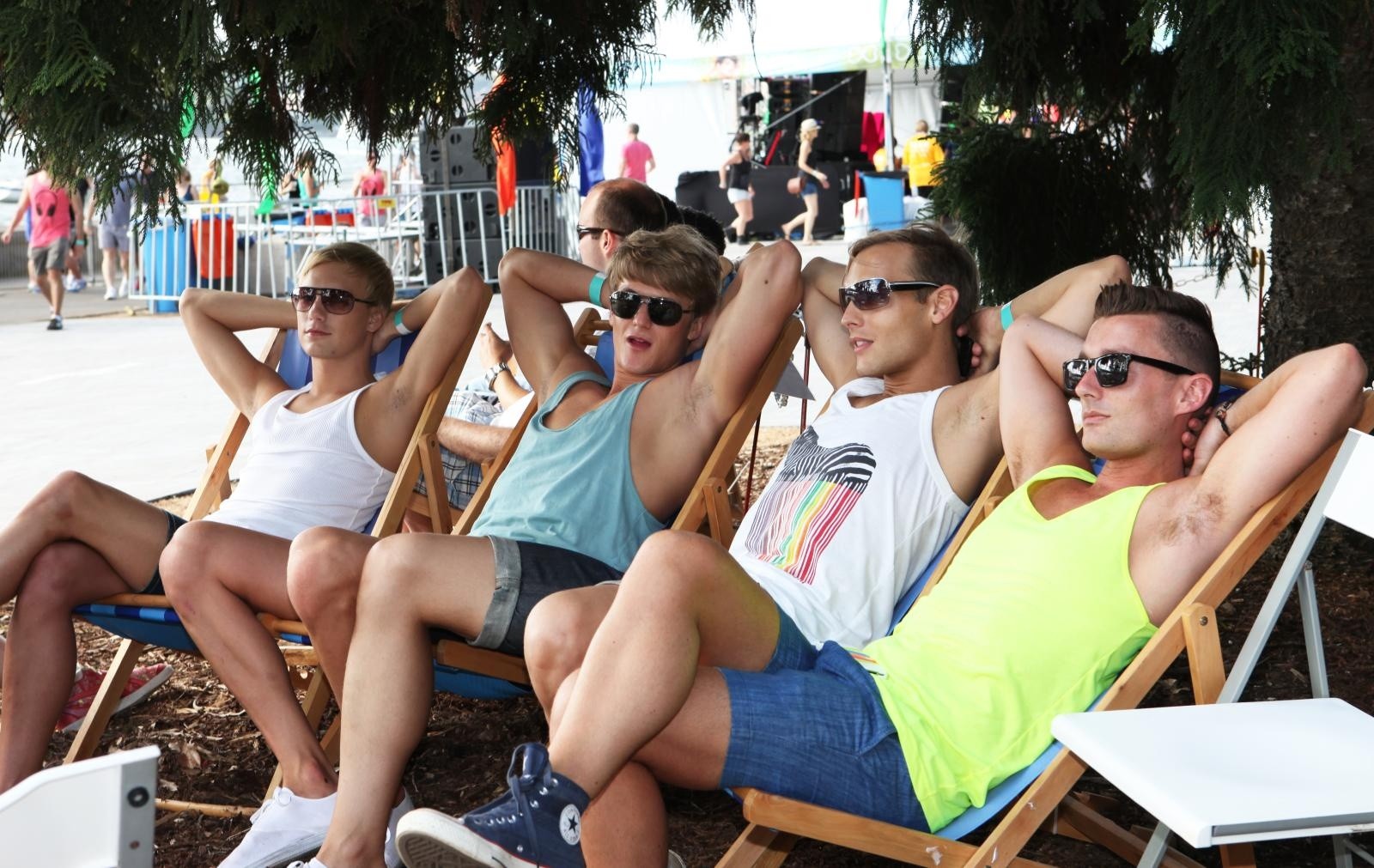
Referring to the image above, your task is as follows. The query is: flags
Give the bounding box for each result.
[483,69,516,216]
[575,73,604,195]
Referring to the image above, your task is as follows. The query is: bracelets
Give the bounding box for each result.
[589,271,609,308]
[1001,299,1013,330]
[1214,399,1233,436]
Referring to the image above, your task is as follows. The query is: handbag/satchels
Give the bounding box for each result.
[786,177,805,194]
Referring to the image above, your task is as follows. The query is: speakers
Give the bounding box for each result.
[421,125,554,287]
[938,63,971,133]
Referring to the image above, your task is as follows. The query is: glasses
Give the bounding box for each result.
[289,287,375,315]
[1063,353,1196,390]
[839,278,943,313]
[609,291,694,327]
[576,224,626,239]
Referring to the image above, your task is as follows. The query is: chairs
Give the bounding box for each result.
[60,283,1372,867]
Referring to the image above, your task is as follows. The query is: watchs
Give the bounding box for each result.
[484,362,510,392]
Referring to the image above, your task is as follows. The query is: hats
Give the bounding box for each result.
[800,119,821,131]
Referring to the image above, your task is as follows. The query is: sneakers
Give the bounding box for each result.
[383,787,416,868]
[216,787,336,868]
[55,663,175,734]
[395,742,591,868]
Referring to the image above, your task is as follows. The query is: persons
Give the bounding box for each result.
[0,242,801,868]
[413,179,725,514]
[395,226,1367,868]
[284,149,399,225]
[1,154,231,331]
[781,119,829,245]
[873,119,948,198]
[719,133,755,245]
[620,123,655,182]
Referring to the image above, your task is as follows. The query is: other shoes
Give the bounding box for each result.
[802,239,821,246]
[104,289,116,300]
[119,284,127,297]
[723,227,732,243]
[47,315,63,329]
[737,236,748,245]
[68,279,87,292]
[780,223,790,241]
[29,284,40,292]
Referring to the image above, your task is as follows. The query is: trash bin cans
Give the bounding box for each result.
[855,169,909,235]
[143,212,234,314]
